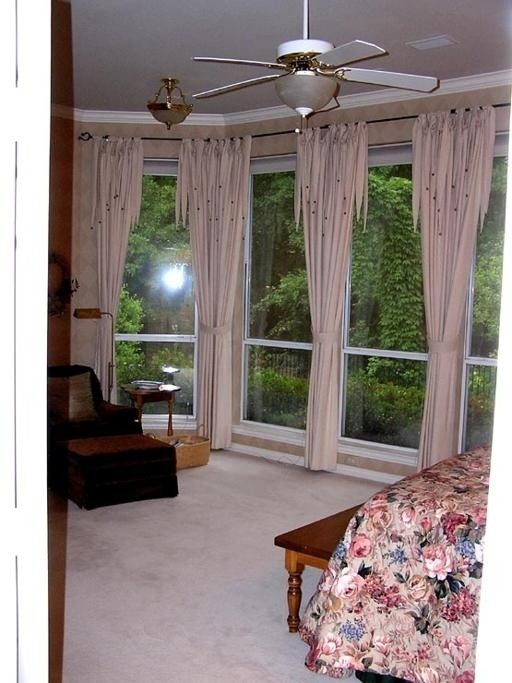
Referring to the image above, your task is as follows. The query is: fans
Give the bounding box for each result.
[191,0,439,100]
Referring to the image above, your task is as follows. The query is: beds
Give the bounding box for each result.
[297,445,492,683]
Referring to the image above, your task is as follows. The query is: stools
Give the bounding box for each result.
[61,436,179,511]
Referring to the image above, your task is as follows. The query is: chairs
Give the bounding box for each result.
[47,364,143,488]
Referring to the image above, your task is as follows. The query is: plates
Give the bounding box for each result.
[131,380,163,390]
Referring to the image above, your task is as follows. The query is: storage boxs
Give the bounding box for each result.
[156,433,210,470]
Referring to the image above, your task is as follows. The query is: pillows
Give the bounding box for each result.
[47,371,100,421]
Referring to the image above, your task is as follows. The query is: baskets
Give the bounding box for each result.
[147,424,211,470]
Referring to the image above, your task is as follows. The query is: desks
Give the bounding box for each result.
[121,384,180,437]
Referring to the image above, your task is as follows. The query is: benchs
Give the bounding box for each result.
[273,503,362,634]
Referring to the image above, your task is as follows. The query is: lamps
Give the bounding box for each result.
[274,72,339,118]
[146,78,194,130]
[74,307,114,403]
